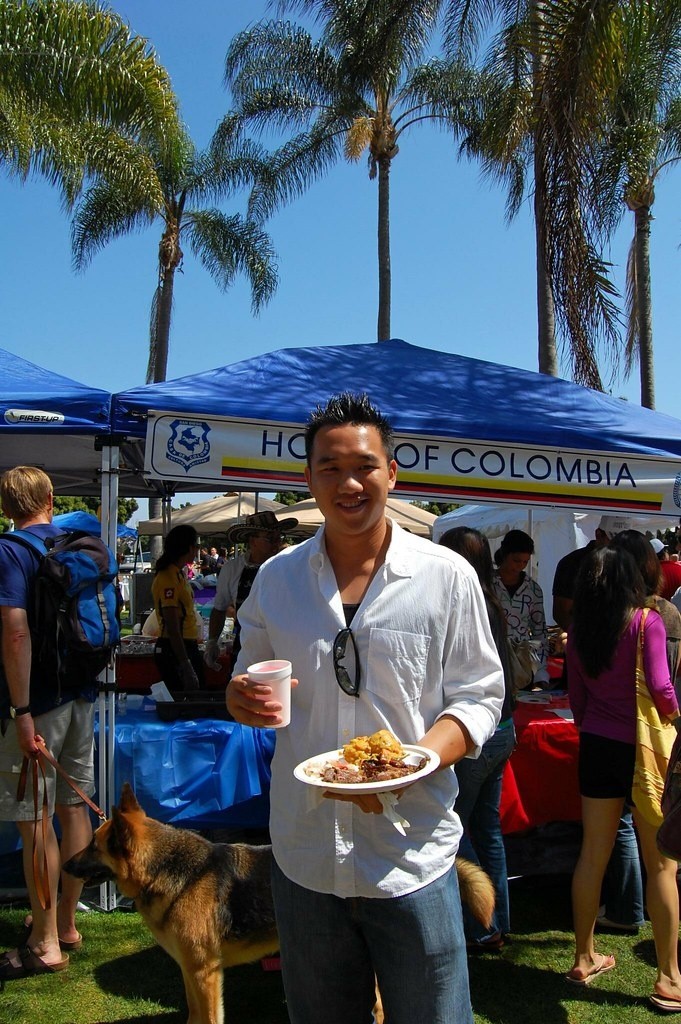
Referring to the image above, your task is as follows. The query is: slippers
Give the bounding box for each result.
[649,992,681,1011]
[0,945,71,979]
[565,952,616,985]
[24,915,82,948]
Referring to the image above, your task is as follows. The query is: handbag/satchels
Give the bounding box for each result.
[506,636,531,690]
[632,607,681,828]
[151,596,204,675]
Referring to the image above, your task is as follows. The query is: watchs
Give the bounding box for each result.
[9,707,29,718]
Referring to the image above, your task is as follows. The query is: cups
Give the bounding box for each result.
[247,660,292,728]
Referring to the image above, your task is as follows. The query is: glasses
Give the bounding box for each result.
[331,627,362,699]
[254,532,282,545]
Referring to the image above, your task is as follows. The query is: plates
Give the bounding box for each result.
[293,745,440,795]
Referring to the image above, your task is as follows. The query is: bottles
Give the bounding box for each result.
[118,693,127,716]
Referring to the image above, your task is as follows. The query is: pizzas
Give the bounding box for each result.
[359,756,429,783]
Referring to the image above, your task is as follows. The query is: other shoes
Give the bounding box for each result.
[594,905,640,935]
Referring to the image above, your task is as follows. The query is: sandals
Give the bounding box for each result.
[468,933,504,950]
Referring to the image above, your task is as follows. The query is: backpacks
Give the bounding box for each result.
[1,527,122,664]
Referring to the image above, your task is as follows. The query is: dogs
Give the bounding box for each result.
[59,782,497,1024]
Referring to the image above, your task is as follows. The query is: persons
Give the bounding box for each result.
[438,527,517,954]
[226,392,505,1024]
[200,546,225,576]
[205,512,299,667]
[0,465,96,979]
[551,516,681,1010]
[150,524,198,716]
[491,530,548,675]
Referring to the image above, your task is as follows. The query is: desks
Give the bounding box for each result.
[545,655,566,677]
[117,651,235,690]
[0,692,275,853]
[500,691,583,830]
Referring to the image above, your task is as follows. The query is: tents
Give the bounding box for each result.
[269,496,438,539]
[50,510,137,540]
[0,347,112,909]
[431,504,681,631]
[108,338,681,910]
[134,496,288,574]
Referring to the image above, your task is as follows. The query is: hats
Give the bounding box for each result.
[226,511,299,544]
[649,539,669,554]
